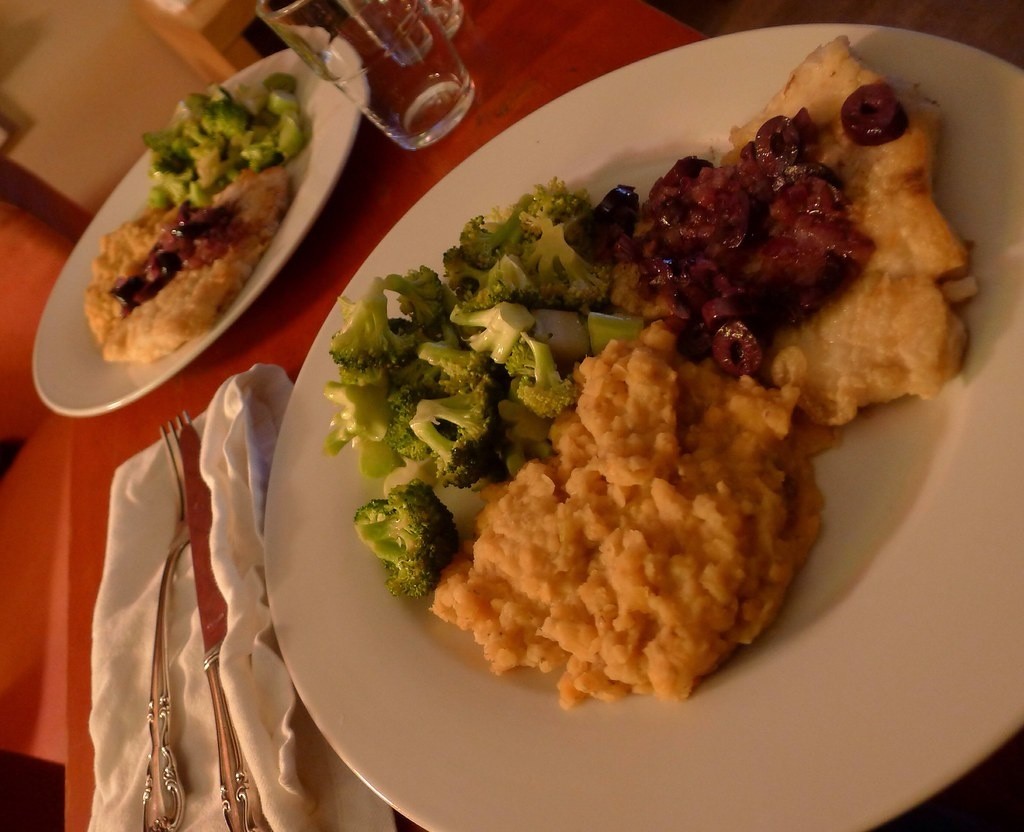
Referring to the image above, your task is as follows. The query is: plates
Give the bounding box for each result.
[266,22,1022,832]
[33,38,368,418]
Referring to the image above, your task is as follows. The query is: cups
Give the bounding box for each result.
[256,0,473,152]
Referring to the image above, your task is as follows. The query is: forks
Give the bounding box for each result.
[142,411,192,831]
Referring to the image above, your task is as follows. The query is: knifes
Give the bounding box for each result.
[179,424,253,832]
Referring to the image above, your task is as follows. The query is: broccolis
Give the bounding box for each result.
[138,71,305,204]
[319,176,639,602]
